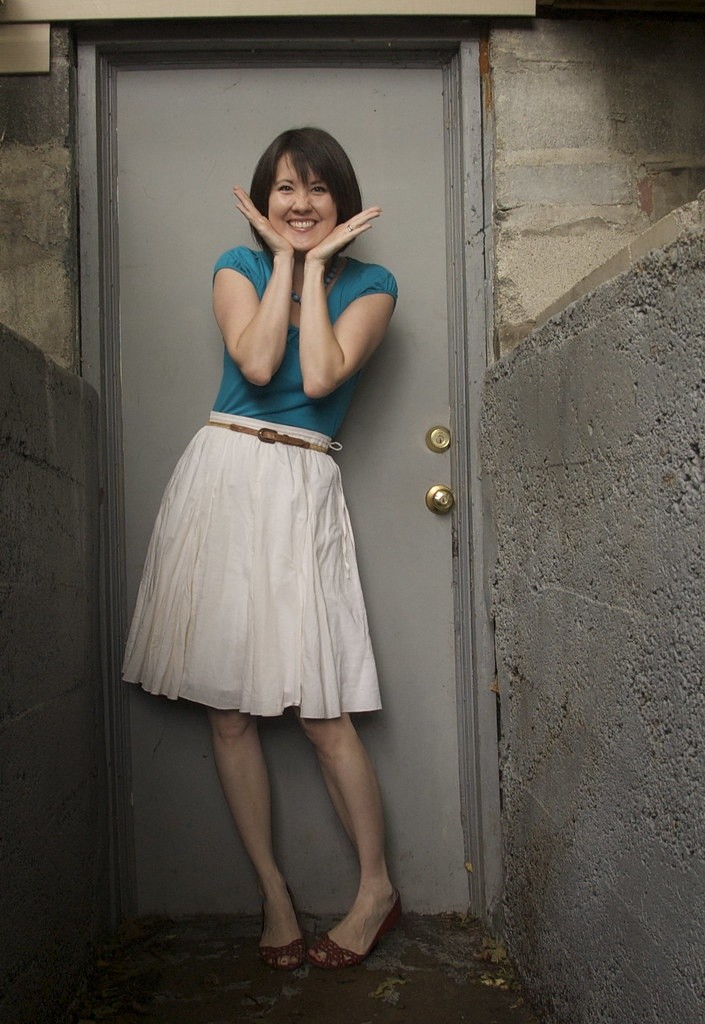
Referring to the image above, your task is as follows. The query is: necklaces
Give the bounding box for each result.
[292,254,340,306]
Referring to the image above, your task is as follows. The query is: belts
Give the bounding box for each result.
[206,421,329,455]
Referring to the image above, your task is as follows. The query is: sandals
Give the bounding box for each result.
[260,882,307,971]
[308,887,401,970]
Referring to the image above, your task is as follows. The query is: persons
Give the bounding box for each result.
[120,132,408,973]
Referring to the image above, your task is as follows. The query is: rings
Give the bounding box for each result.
[348,225,354,232]
